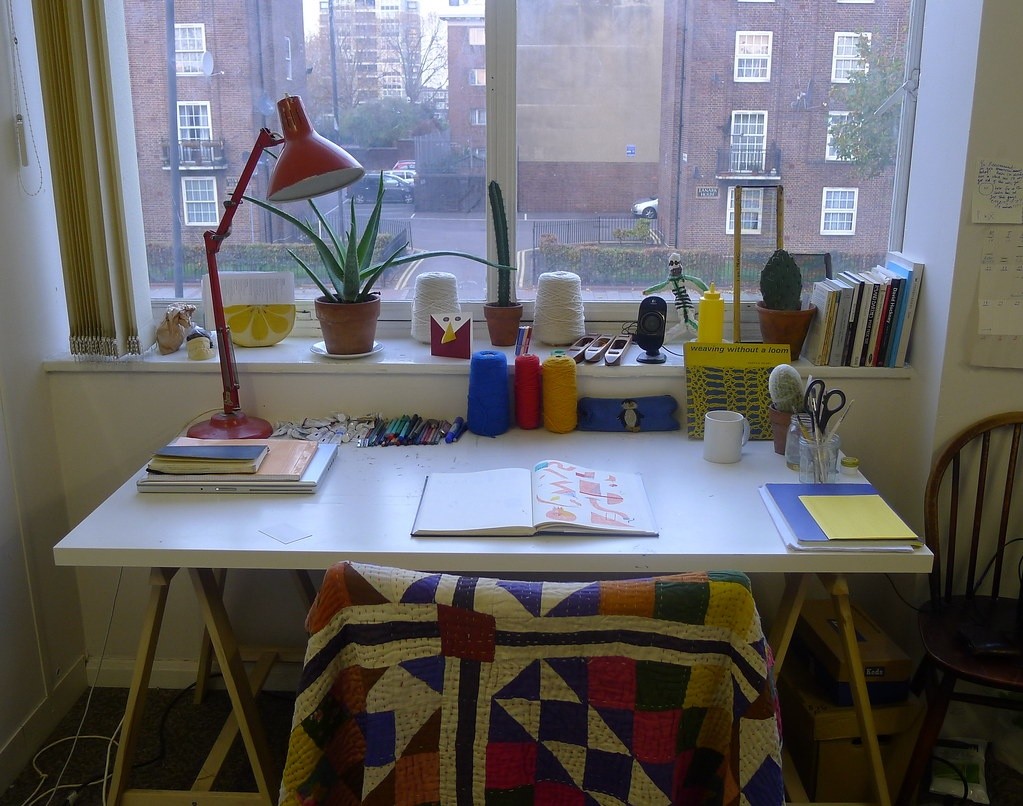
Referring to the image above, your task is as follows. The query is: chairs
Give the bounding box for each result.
[279,558,787,806]
[895,408,1023,806]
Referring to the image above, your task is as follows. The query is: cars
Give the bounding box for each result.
[630,196,658,218]
[346,160,417,204]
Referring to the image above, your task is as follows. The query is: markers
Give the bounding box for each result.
[445,416,464,443]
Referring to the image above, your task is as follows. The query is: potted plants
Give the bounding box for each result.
[227,169,519,351]
[754,246,816,361]
[480,177,527,346]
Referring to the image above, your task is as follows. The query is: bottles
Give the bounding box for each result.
[698,281,725,343]
[785,414,818,472]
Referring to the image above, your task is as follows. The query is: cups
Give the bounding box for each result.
[798,431,840,484]
[703,411,750,464]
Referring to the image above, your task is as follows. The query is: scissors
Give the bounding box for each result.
[804,380,847,482]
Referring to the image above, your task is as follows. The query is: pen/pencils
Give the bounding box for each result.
[453,421,469,442]
[515,326,532,355]
[365,414,446,446]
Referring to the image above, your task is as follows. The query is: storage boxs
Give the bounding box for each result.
[782,667,928,806]
[798,598,910,703]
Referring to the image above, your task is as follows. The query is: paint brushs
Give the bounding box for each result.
[811,409,821,441]
[826,400,855,442]
[812,388,818,413]
[791,405,811,440]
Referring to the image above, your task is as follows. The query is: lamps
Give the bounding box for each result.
[190,88,369,440]
[692,166,703,179]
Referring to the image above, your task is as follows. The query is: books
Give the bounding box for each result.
[802,252,924,368]
[147,436,318,480]
[410,459,659,537]
[764,483,924,551]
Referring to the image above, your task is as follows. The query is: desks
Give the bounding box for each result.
[49,416,936,806]
